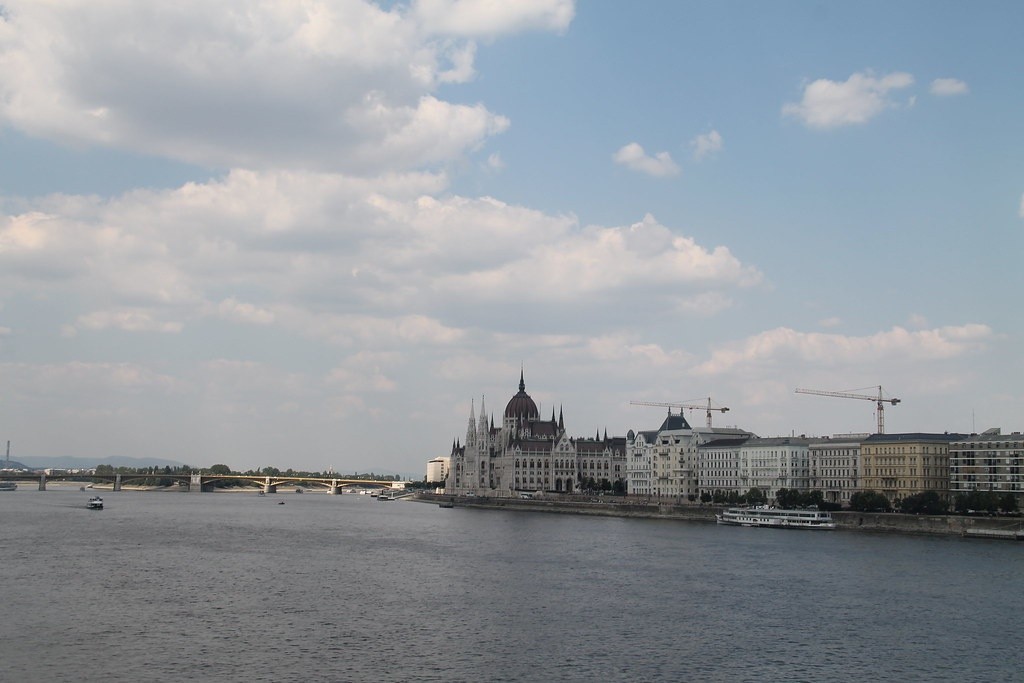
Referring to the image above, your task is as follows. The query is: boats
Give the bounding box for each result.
[86,496,103,510]
[713,505,837,531]
[258,490,265,497]
[0,483,19,491]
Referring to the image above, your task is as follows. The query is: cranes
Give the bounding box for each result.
[794,385,901,435]
[628,397,730,428]
